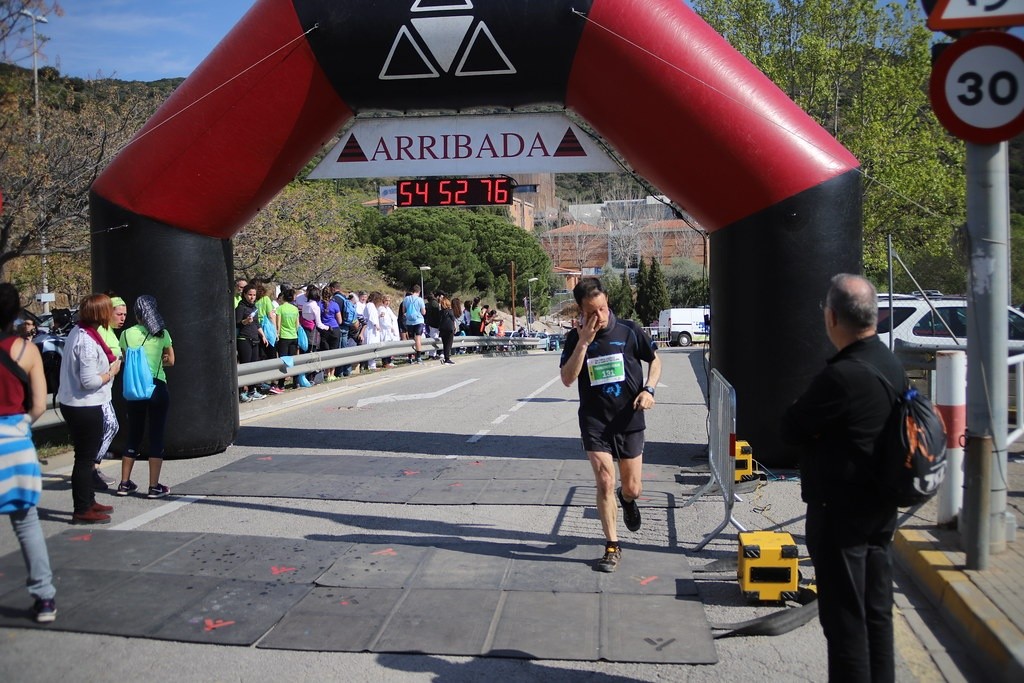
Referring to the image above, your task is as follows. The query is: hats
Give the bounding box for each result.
[108,296,126,308]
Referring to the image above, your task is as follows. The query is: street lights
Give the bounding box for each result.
[420,266,431,300]
[528,277,539,332]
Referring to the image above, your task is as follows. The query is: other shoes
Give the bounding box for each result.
[417,358,423,363]
[444,359,454,364]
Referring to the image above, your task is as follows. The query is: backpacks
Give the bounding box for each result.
[334,294,357,326]
[119,329,162,400]
[408,295,422,321]
[838,357,947,507]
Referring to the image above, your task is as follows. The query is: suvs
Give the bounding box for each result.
[875,291,1024,407]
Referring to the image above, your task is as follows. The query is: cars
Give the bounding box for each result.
[14,307,77,372]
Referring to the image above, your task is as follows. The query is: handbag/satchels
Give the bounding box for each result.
[261,314,276,346]
[308,329,320,348]
[298,325,307,351]
[248,338,260,347]
[300,316,315,331]
[439,308,454,333]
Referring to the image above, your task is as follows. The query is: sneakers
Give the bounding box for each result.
[34,599,58,623]
[617,486,641,532]
[116,480,138,495]
[597,544,622,573]
[148,484,171,499]
[72,508,111,524]
[90,502,114,514]
[92,471,108,493]
[238,358,414,402]
[96,467,115,485]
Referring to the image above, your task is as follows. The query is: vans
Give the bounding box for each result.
[658,308,711,346]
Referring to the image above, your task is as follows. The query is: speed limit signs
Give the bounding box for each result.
[929,32,1024,142]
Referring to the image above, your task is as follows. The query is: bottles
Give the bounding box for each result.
[247,308,259,317]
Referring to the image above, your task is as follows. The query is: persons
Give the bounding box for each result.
[48,317,68,337]
[55,293,121,524]
[559,276,662,572]
[94,297,126,485]
[517,327,525,350]
[0,282,58,622]
[118,294,175,497]
[17,317,49,344]
[234,278,507,403]
[781,272,910,683]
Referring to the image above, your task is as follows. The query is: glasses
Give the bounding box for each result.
[818,298,838,320]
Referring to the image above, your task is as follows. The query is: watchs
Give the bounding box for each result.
[105,372,114,383]
[643,386,654,397]
